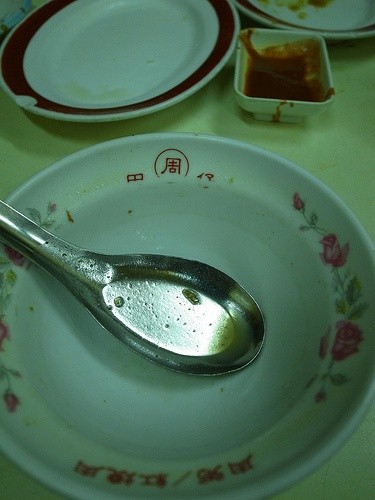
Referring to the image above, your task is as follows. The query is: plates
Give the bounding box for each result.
[0,0,240,123]
[234,27,335,126]
[0,132,375,500]
[232,0,375,40]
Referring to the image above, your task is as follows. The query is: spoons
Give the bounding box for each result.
[0,197,265,377]
